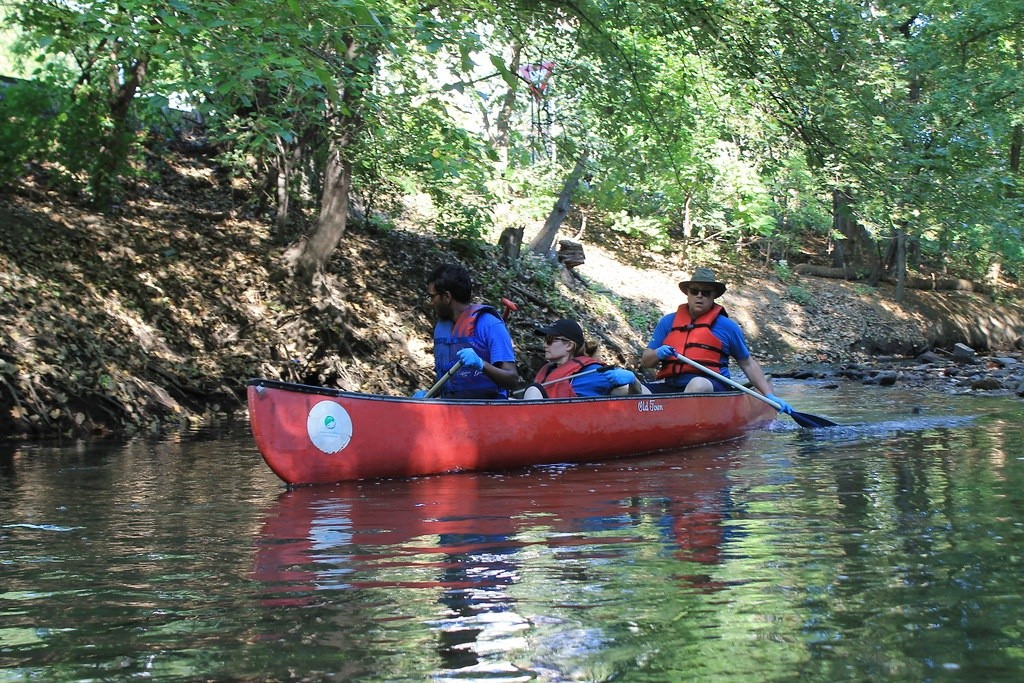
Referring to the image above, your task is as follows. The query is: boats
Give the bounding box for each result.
[243,375,774,487]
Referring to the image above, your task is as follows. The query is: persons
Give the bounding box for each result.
[524,318,643,400]
[641,267,797,414]
[413,263,519,401]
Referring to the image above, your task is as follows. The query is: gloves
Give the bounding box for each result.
[412,391,433,398]
[456,348,483,372]
[602,367,635,387]
[766,393,796,415]
[656,345,674,360]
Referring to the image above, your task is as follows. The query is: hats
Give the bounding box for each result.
[535,319,584,346]
[679,269,726,299]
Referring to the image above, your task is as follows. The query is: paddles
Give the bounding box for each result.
[676,351,839,427]
[423,357,462,398]
[501,296,518,322]
[512,364,616,396]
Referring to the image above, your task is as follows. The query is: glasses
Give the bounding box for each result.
[427,291,444,300]
[687,286,715,297]
[543,335,571,345]
